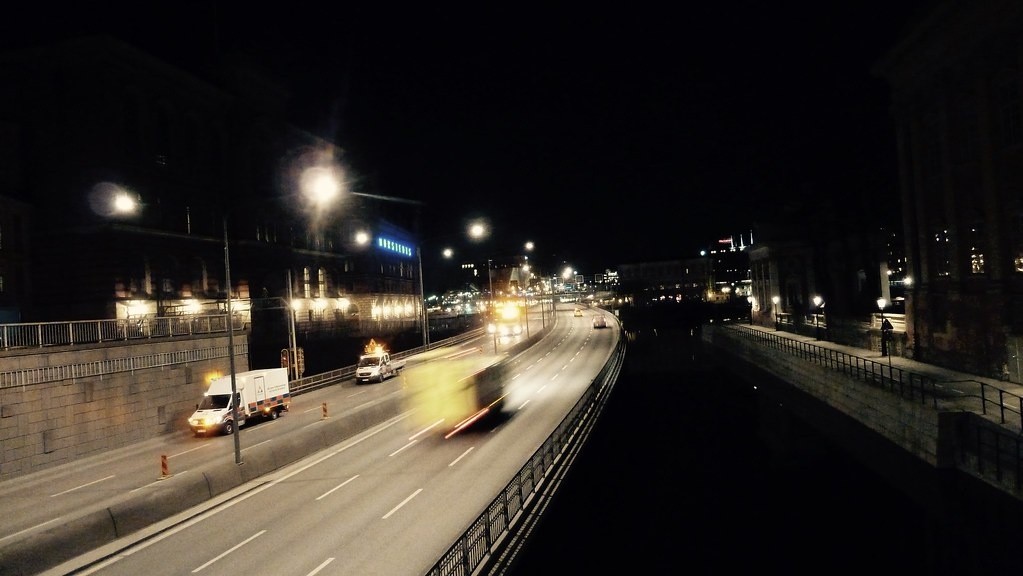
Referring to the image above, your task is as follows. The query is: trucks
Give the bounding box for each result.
[189,367,290,435]
[355,353,407,384]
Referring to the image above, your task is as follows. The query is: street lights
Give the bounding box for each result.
[523,239,534,343]
[286,167,339,380]
[467,220,498,356]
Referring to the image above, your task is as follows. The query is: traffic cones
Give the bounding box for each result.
[318,401,332,421]
[156,454,175,481]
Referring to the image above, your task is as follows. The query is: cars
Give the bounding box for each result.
[592,315,606,327]
[574,308,582,317]
